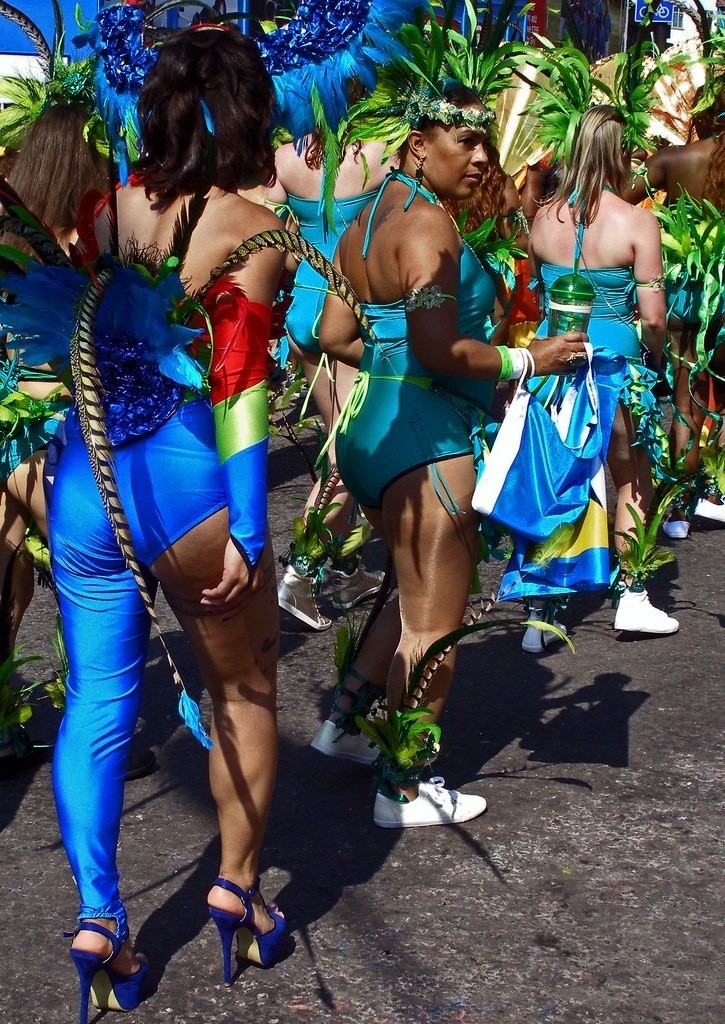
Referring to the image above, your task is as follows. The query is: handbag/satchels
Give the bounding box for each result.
[470,340,602,538]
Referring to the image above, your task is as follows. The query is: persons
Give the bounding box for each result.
[618,103,722,540]
[0,1,588,1024]
[494,104,679,653]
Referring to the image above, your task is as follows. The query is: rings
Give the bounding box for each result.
[569,354,587,369]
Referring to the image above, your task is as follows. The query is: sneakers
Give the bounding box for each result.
[520,615,568,652]
[310,719,380,764]
[372,777,488,828]
[276,565,332,631]
[613,590,679,635]
[329,564,385,609]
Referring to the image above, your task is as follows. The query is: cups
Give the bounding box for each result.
[546,273,596,376]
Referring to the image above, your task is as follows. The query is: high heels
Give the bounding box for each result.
[62,923,149,1024]
[207,879,290,989]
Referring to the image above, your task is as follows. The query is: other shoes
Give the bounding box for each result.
[693,495,725,522]
[0,738,45,782]
[663,514,691,538]
[46,712,157,779]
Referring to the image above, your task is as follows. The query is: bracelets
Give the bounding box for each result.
[507,348,523,381]
[495,345,511,384]
